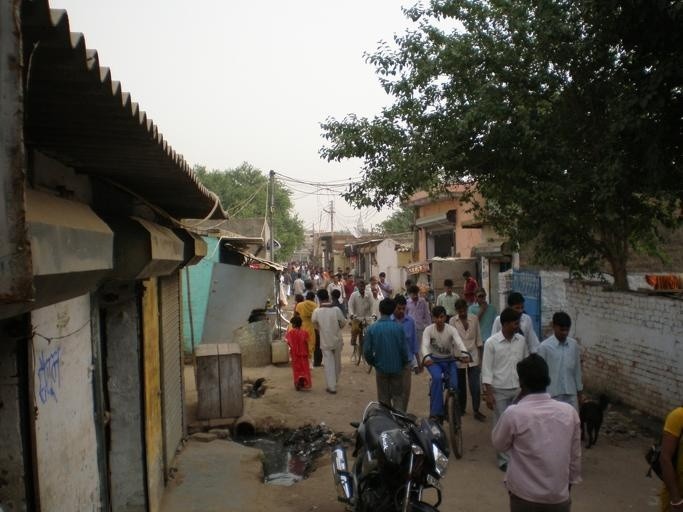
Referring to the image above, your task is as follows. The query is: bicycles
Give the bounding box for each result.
[346,315,378,376]
[419,350,476,460]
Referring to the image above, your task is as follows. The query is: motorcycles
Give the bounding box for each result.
[327,395,450,511]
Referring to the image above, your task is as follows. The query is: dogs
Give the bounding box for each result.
[577,391,608,449]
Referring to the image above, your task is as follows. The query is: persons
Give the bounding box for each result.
[276,259,588,472]
[657,403,682,511]
[489,353,584,511]
